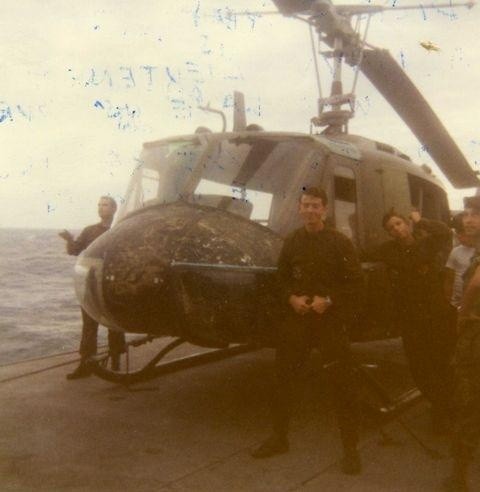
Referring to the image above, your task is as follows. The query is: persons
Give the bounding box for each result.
[364,197,480,492]
[253,189,364,476]
[59,194,128,380]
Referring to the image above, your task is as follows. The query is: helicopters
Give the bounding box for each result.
[74,0,480,418]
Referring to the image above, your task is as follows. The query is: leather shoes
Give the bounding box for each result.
[251,434,289,458]
[342,450,360,472]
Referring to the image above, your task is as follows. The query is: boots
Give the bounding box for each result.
[67,356,91,379]
